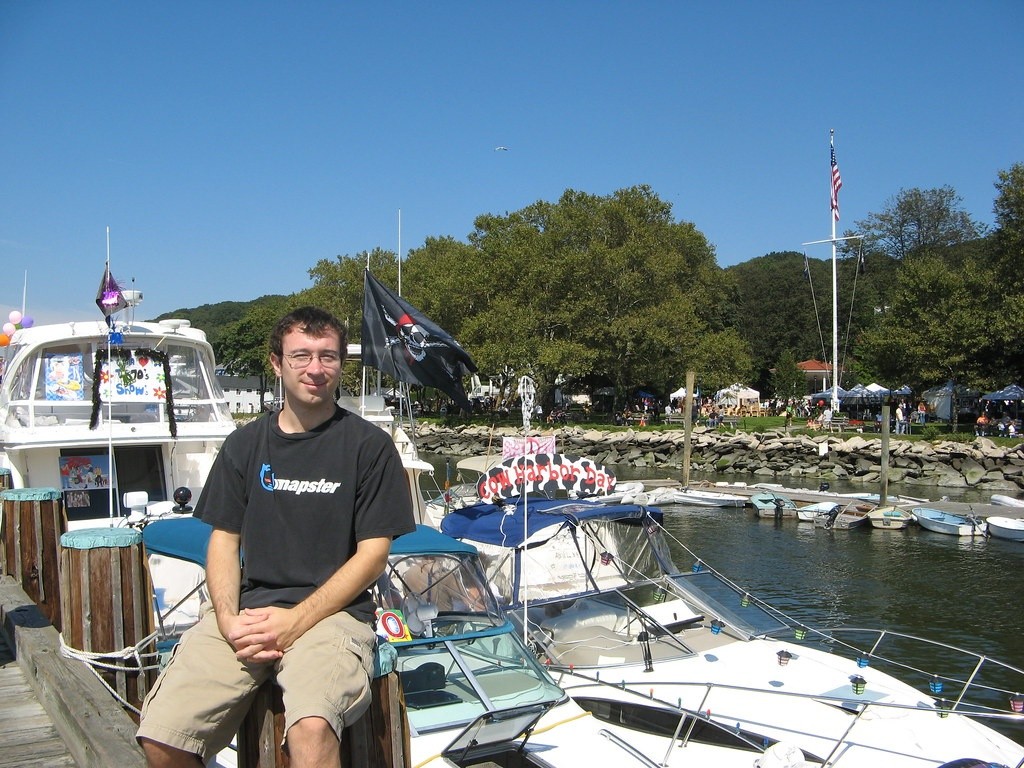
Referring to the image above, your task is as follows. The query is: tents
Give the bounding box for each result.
[717,382,760,411]
[982,383,1024,419]
[923,380,986,421]
[631,389,653,398]
[812,383,911,420]
[670,387,699,403]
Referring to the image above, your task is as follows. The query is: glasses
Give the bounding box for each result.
[282,351,341,366]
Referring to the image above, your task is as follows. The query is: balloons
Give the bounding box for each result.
[0,310,33,348]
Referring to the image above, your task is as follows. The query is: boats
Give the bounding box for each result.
[812,501,879,531]
[909,505,989,538]
[795,501,846,529]
[984,515,1024,543]
[867,506,918,530]
[565,480,681,510]
[2,225,1024,768]
[749,489,799,518]
[673,481,750,509]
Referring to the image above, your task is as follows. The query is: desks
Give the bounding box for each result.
[723,409,728,415]
[741,409,755,417]
[751,410,766,417]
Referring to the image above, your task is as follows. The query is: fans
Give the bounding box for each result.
[400,591,438,638]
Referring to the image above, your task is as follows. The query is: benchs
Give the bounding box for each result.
[547,412,576,424]
[706,418,738,428]
[950,521,965,524]
[829,415,864,434]
[847,510,866,516]
[855,505,872,510]
[930,517,945,521]
[626,414,652,426]
[758,498,774,501]
[883,512,901,516]
[664,415,684,424]
[720,408,772,417]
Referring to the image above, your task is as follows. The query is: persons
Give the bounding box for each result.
[614,397,727,428]
[136,302,416,768]
[740,399,812,426]
[547,409,566,425]
[401,552,493,613]
[817,397,926,435]
[497,404,509,412]
[973,410,1020,438]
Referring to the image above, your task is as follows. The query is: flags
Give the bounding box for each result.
[361,268,480,416]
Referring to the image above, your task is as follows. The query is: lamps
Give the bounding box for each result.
[710,619,726,635]
[652,586,666,601]
[794,625,808,640]
[933,700,953,718]
[691,560,704,573]
[776,651,792,666]
[1009,694,1024,712]
[928,676,945,693]
[599,552,614,566]
[647,521,660,534]
[739,594,753,608]
[850,676,867,695]
[856,653,872,668]
